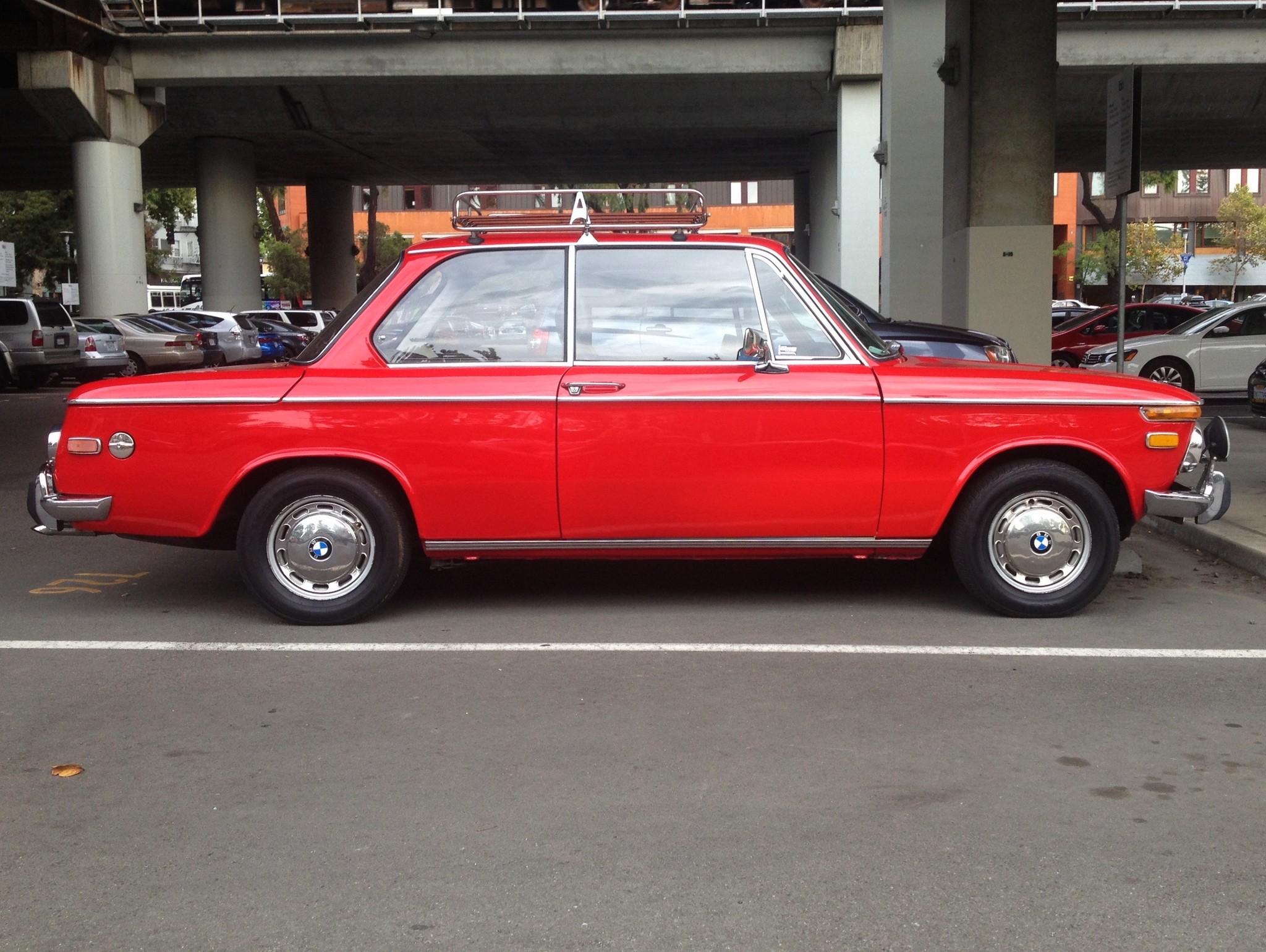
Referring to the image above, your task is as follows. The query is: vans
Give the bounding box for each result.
[0,292,81,388]
[240,310,338,335]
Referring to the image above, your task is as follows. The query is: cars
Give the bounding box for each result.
[71,310,317,378]
[1049,292,1266,418]
[24,228,1237,627]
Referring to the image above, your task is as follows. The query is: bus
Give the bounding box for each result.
[178,273,284,307]
